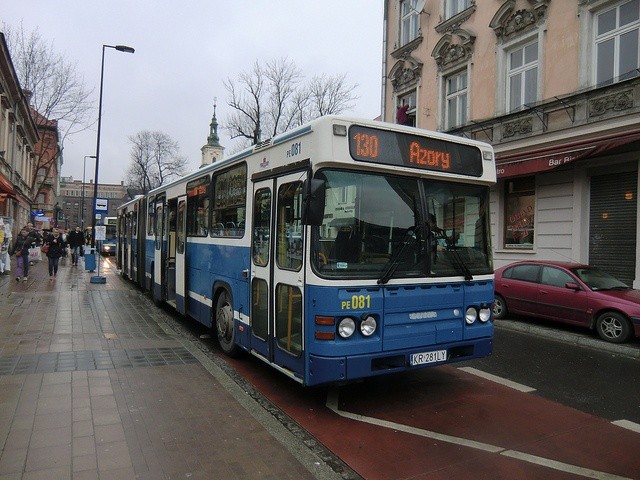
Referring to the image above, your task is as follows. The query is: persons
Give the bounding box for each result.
[10,221,32,281]
[27,223,42,266]
[397,104,412,126]
[43,227,63,280]
[67,225,85,267]
[428,213,459,246]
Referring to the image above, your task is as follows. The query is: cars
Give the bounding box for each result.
[493,260,640,344]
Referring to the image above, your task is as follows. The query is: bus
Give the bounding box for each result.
[85,227,95,245]
[117,114,498,388]
[96,217,117,255]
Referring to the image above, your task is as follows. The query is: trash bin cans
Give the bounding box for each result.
[83,248,96,272]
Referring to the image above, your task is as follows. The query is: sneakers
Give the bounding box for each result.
[75,263,78,266]
[23,277,27,281]
[49,276,53,280]
[72,263,75,266]
[54,275,57,279]
[15,276,20,281]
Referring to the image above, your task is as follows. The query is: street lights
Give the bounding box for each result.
[90,44,135,252]
[81,155,97,231]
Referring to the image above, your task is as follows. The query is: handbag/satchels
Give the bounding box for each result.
[80,246,85,256]
[28,246,42,261]
[41,241,48,253]
[15,250,21,256]
